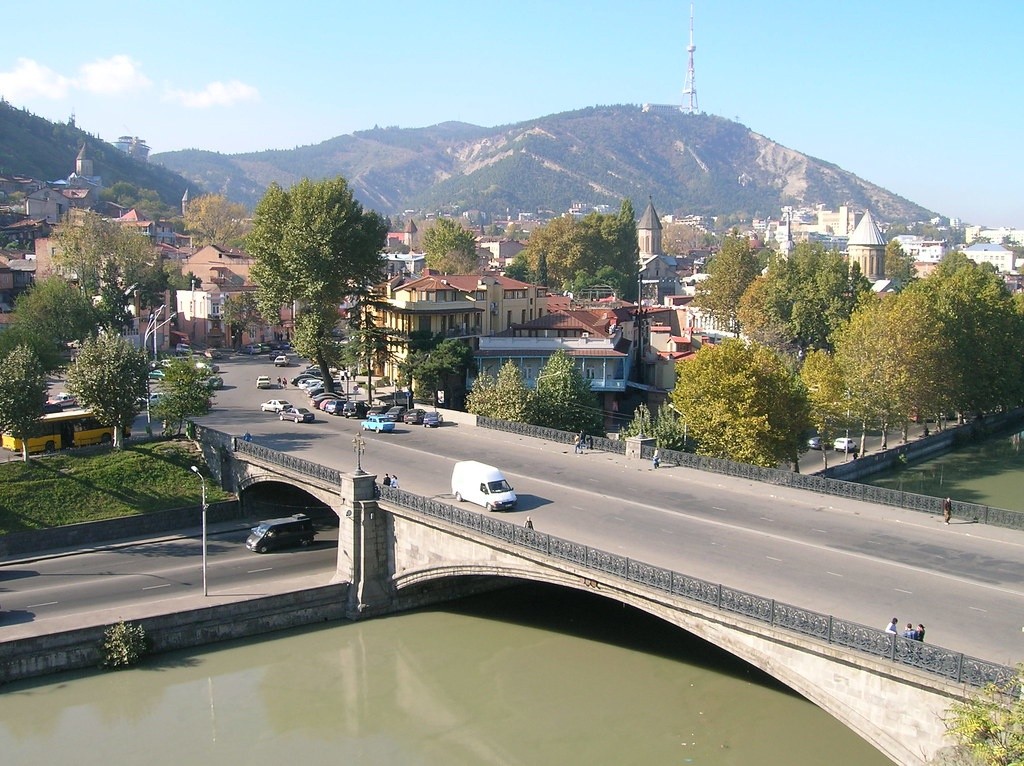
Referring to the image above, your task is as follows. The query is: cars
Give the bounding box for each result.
[935,409,956,419]
[138,327,444,433]
[48,392,78,408]
[911,409,937,424]
[808,436,828,450]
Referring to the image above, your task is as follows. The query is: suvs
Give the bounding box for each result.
[833,438,857,452]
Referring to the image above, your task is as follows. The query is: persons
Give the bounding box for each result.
[652,447,660,469]
[161,419,172,436]
[275,376,287,389]
[853,445,859,460]
[243,430,253,442]
[439,414,444,427]
[943,497,952,526]
[936,418,941,432]
[574,430,593,454]
[884,617,925,662]
[268,531,273,537]
[383,473,399,489]
[523,516,533,544]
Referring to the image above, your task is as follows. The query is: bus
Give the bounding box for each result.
[3,408,133,453]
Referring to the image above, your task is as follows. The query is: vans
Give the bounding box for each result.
[246,513,316,554]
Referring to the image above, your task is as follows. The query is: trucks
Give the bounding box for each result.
[451,459,518,512]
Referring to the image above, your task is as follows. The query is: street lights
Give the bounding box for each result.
[190,465,208,599]
[142,305,179,440]
